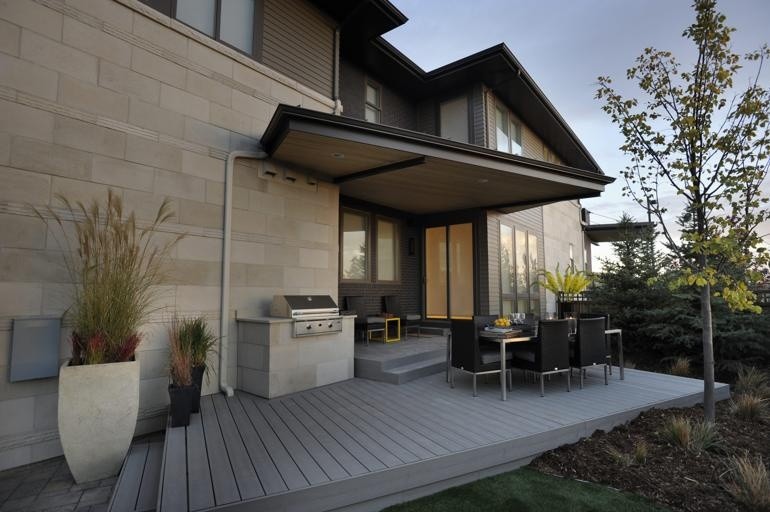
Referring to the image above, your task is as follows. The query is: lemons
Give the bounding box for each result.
[495,319,510,326]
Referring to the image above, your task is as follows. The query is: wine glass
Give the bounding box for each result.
[509,312,525,324]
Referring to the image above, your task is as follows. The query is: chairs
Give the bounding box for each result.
[472,315,499,383]
[345,295,386,346]
[383,295,421,341]
[450,319,512,397]
[571,313,612,379]
[509,313,534,327]
[512,319,571,395]
[567,317,608,389]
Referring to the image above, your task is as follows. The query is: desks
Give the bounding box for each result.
[446,328,624,401]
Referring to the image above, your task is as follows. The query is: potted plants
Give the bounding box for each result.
[27,187,185,488]
[165,331,195,428]
[178,317,224,412]
